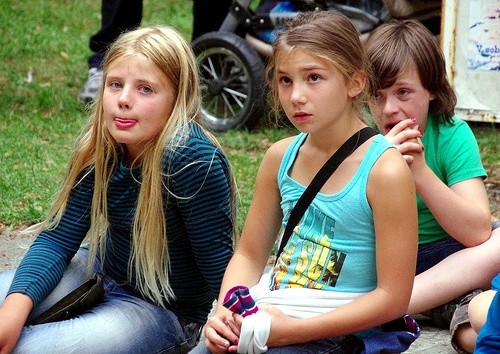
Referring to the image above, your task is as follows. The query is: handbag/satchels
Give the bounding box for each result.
[27,271,106,326]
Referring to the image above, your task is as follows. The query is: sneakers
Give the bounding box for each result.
[75,68,108,106]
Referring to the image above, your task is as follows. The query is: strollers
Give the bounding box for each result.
[188,0,389,133]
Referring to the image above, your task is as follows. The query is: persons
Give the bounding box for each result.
[79,0,232,107]
[363,20,500,354]
[0,26,237,354]
[186,11,418,354]
